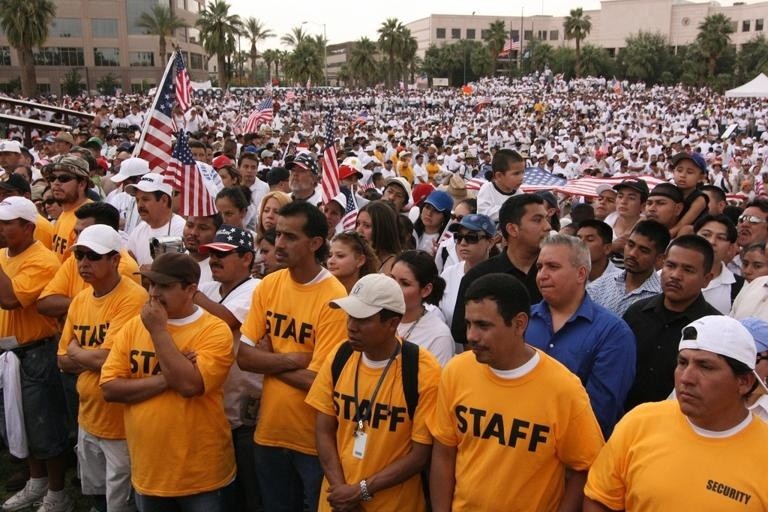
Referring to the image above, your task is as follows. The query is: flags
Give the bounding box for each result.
[131,53,176,174]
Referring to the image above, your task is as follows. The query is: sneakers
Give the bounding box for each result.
[35,481,75,511]
[2,478,50,511]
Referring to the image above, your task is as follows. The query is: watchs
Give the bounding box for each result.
[359,479,372,503]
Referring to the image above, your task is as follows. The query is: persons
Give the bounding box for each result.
[0,196,72,512]
[305,272,443,512]
[422,271,605,512]
[34,202,142,459]
[171,66,768,440]
[0,91,171,268]
[582,314,768,512]
[236,198,349,512]
[56,224,150,512]
[99,250,237,512]
[191,224,264,511]
